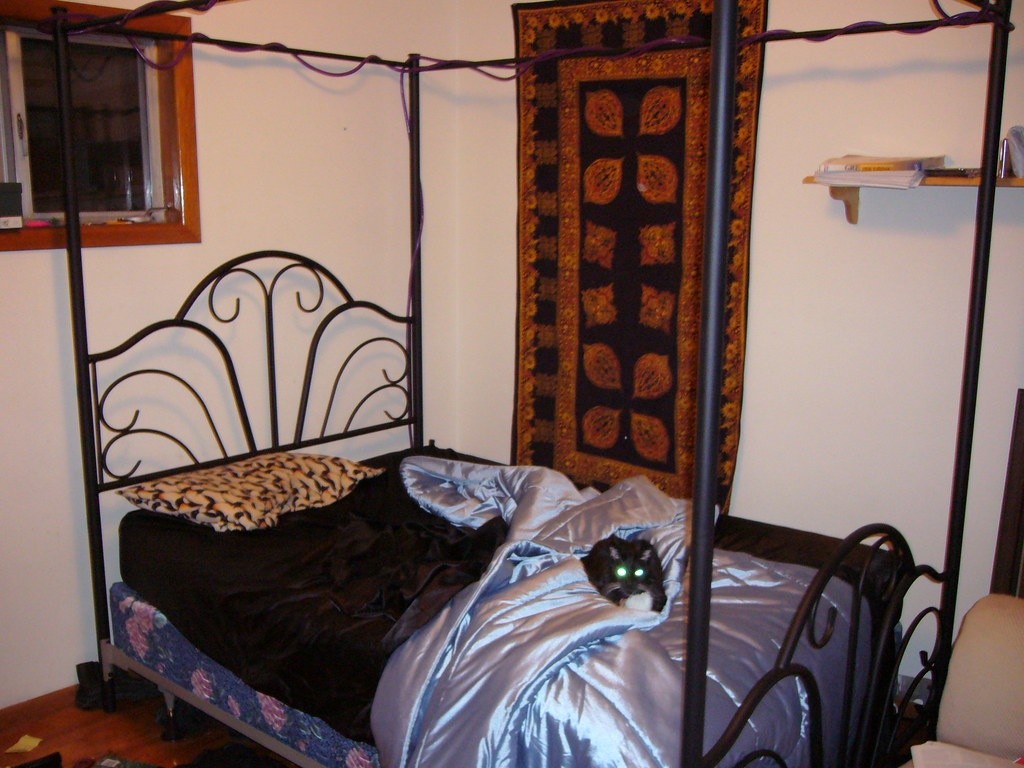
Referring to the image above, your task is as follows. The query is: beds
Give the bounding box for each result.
[49,0,1016,768]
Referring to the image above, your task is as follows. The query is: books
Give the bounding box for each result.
[813,154,943,189]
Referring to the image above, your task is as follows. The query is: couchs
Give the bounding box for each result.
[897,593,1024,768]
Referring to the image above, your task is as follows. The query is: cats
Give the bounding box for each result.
[580,533,668,613]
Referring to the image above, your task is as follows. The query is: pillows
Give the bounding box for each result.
[114,453,386,534]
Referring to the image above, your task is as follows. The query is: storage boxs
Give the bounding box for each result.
[0,183,24,231]
